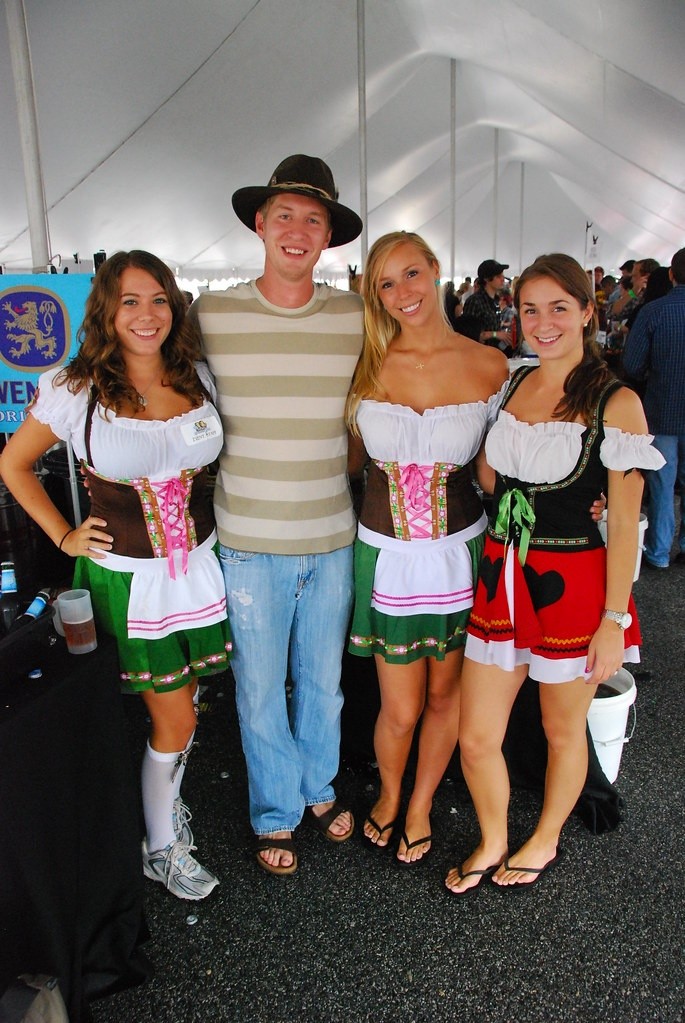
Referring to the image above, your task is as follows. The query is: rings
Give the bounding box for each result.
[614,670,618,676]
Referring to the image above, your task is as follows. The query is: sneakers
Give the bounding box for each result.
[140,836,220,903]
[171,795,196,852]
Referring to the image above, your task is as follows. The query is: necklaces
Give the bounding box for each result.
[396,336,447,370]
[138,370,164,406]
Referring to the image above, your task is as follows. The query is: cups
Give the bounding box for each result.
[52,589,97,655]
[498,341,508,350]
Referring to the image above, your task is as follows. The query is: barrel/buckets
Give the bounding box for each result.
[522,341,539,357]
[587,667,637,786]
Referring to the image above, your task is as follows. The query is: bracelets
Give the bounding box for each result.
[59,528,76,552]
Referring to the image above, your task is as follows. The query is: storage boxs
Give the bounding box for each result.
[0,600,55,689]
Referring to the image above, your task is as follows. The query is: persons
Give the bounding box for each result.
[444,252,665,897]
[188,154,369,877]
[624,246,685,569]
[445,258,675,430]
[0,250,233,902]
[343,231,606,866]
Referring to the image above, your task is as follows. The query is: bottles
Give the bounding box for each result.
[0,561,23,639]
[6,591,51,638]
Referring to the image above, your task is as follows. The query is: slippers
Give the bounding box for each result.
[442,845,516,897]
[489,845,565,892]
[361,800,400,853]
[393,808,433,866]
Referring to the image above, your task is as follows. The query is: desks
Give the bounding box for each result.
[0,598,150,1000]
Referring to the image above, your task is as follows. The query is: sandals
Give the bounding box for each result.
[303,802,356,843]
[252,830,299,874]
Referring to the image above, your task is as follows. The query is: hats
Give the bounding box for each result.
[231,153,363,248]
[478,260,509,280]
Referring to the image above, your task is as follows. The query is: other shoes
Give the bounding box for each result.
[643,552,669,567]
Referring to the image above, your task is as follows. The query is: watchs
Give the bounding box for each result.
[601,608,632,629]
